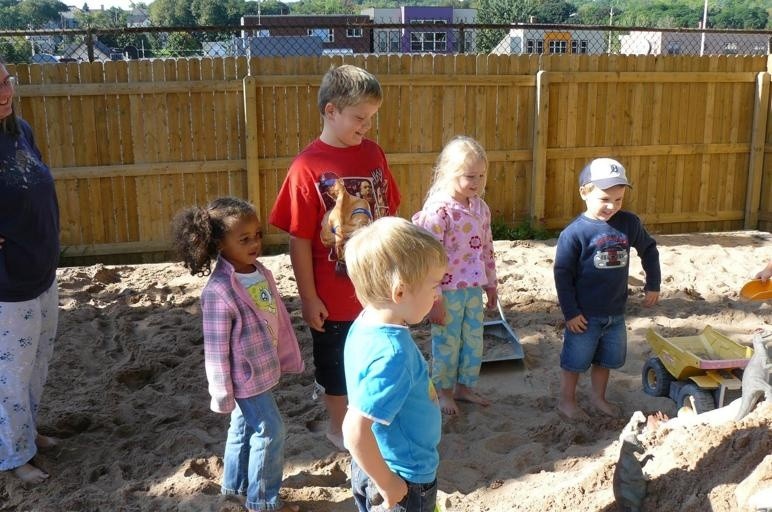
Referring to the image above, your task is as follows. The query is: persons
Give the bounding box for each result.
[336,217,451,510]
[269,65,403,455]
[554,156,663,420]
[317,171,375,276]
[1,63,58,486]
[411,135,496,414]
[357,179,375,204]
[374,185,388,216]
[172,198,306,510]
[753,261,771,285]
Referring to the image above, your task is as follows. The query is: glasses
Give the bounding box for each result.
[0,76,16,89]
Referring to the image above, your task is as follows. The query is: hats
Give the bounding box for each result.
[579,155,633,189]
